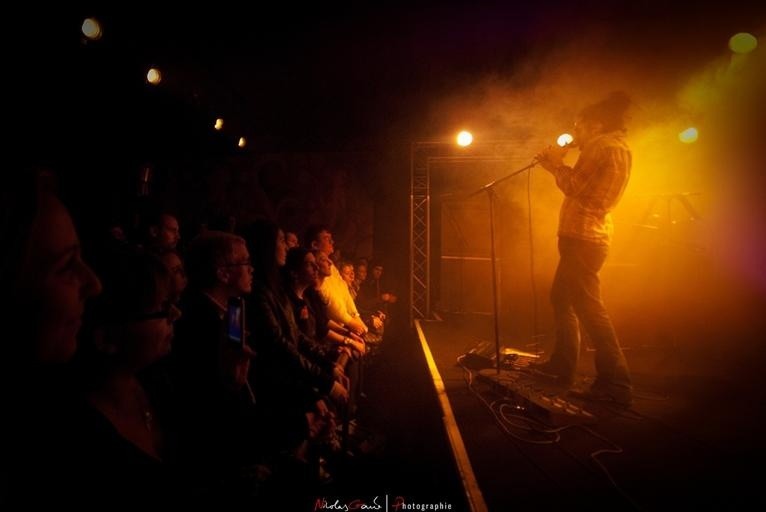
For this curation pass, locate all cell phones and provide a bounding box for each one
[227,295,245,359]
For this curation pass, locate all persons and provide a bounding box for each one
[1,178,399,511]
[531,92,636,406]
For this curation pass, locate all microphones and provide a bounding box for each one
[562,139,579,150]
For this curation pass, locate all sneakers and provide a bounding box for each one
[568,384,632,403]
[523,361,573,383]
